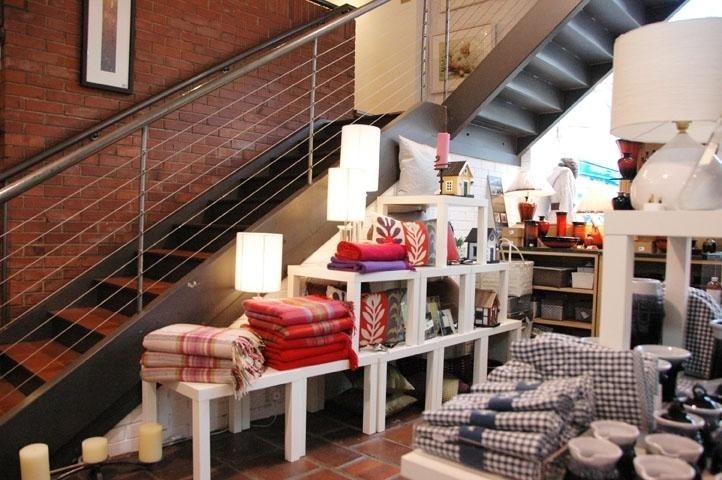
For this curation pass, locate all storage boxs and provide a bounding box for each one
[532,265,594,323]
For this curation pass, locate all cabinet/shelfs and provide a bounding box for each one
[495,248,599,337]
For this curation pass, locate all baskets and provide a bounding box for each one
[540,261,597,326]
[475,238,534,297]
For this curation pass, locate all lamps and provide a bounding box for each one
[325,122,381,242]
[607,15,722,210]
[504,166,557,222]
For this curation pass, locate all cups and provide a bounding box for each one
[564,417,708,480]
[633,345,692,406]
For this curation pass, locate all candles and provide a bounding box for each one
[18,421,165,480]
[435,132,450,168]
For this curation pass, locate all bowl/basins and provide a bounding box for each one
[540,236,580,248]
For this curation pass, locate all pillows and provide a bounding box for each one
[396,133,441,194]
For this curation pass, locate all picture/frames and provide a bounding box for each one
[428,21,498,96]
[79,0,137,97]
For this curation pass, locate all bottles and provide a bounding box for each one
[537,216,550,236]
[555,211,567,237]
[572,222,586,244]
[652,384,722,473]
[523,219,539,248]
[705,277,721,306]
